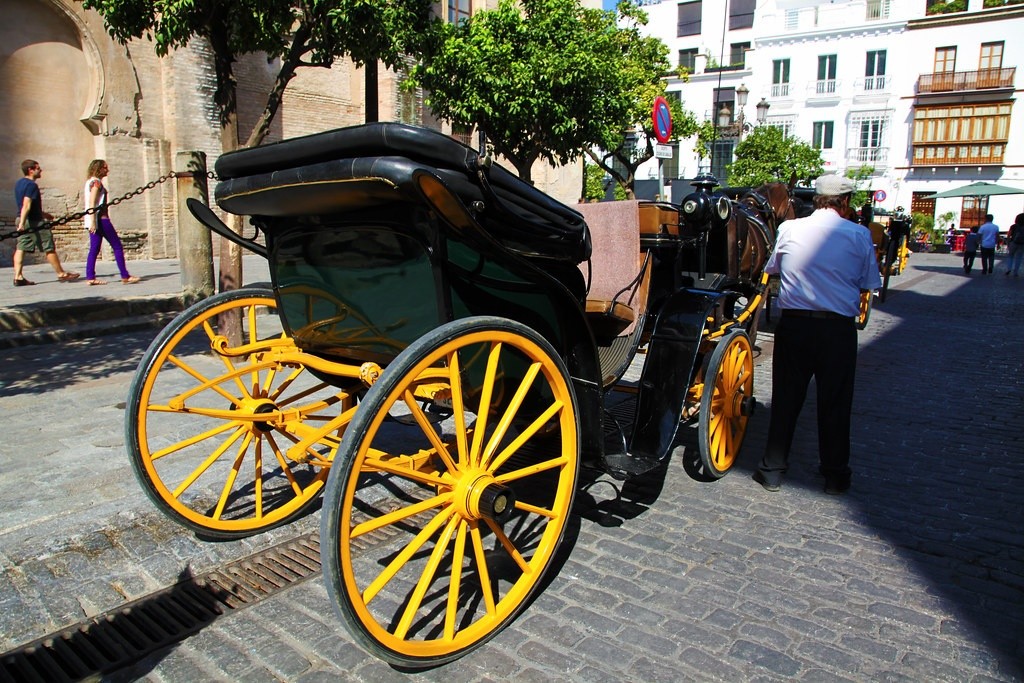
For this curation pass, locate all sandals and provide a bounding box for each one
[87,279,107,285]
[121,276,140,284]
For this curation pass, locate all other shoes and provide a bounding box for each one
[826,485,846,495]
[988,267,992,273]
[1005,268,1011,275]
[754,469,781,491]
[982,270,986,274]
[1014,273,1019,276]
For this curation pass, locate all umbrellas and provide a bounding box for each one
[921,182,1024,250]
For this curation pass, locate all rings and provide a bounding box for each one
[94,229,95,231]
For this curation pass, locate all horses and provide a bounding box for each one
[702,170,799,352]
[868,222,888,274]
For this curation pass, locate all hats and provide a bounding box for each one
[814,174,856,194]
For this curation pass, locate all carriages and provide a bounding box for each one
[121,117,912,677]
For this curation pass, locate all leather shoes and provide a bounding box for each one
[58,272,80,282]
[14,277,35,286]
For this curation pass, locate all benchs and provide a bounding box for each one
[214,122,884,392]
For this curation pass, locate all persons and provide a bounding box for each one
[946,224,956,251]
[1003,213,1024,276]
[753,174,885,495]
[84,160,141,286]
[963,226,979,274]
[13,160,80,287]
[977,214,1001,275]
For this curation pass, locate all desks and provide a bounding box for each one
[942,234,954,250]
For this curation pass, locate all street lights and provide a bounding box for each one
[734,82,772,147]
